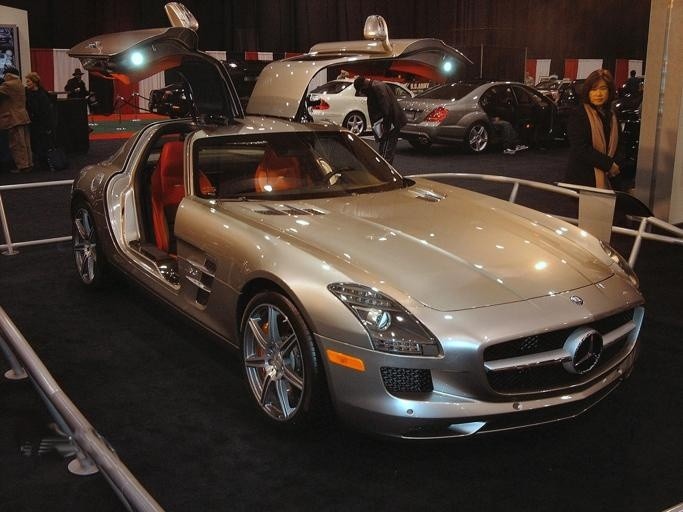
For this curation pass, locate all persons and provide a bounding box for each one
[522,71,535,88]
[0,66,90,174]
[573,68,628,192]
[628,70,638,83]
[353,75,408,166]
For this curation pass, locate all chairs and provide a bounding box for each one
[254,155,315,194]
[147,139,217,261]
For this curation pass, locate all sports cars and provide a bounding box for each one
[65,1,646,444]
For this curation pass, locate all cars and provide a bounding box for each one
[148,58,273,119]
[530,75,644,167]
[305,77,414,137]
[396,82,575,153]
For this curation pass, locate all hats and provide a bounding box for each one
[73,69,84,76]
[3,67,19,76]
[354,77,361,96]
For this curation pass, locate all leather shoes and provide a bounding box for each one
[10,167,32,174]
